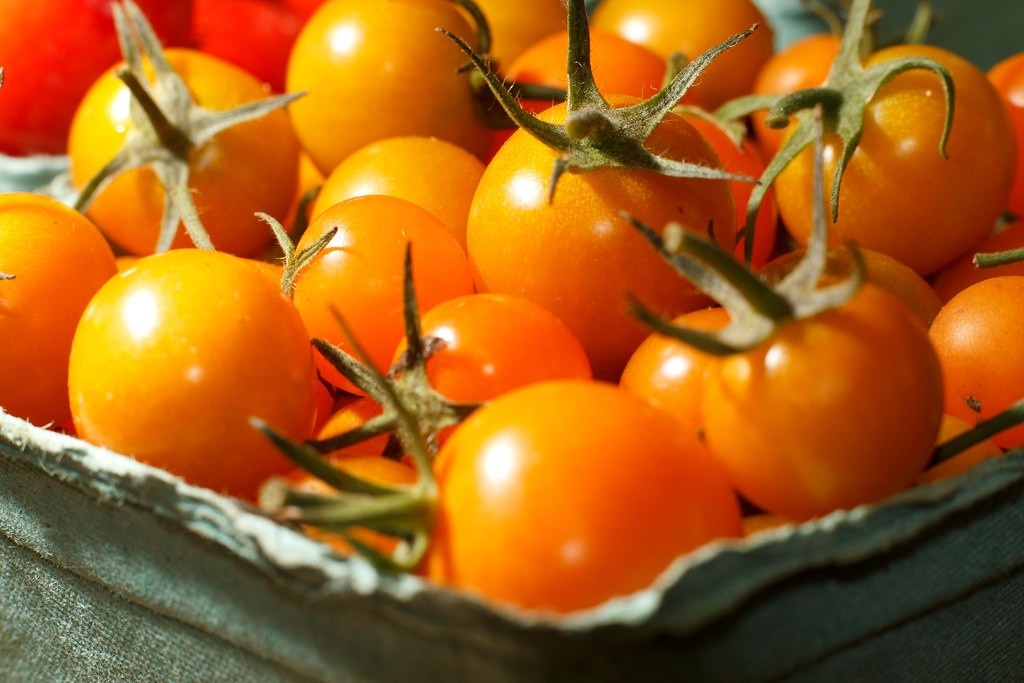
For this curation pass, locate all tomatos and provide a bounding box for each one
[3,0,1024,616]
